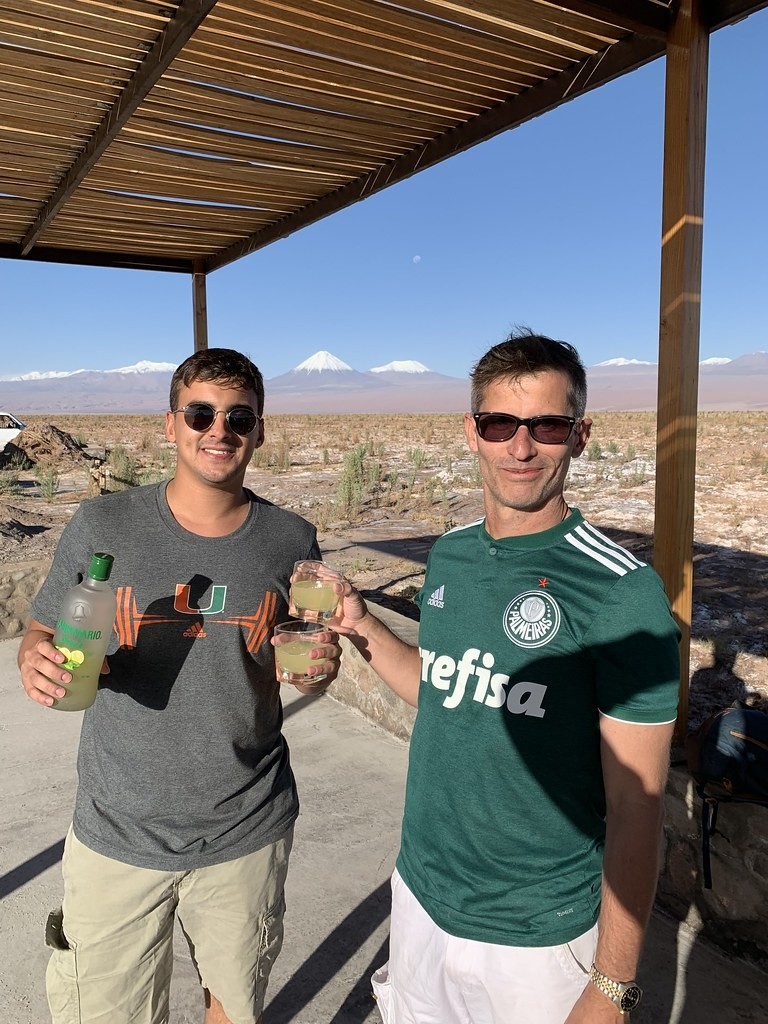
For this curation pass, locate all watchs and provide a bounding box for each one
[586,963,643,1014]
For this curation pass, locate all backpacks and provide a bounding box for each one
[686,700,768,891]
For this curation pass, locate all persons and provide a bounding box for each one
[286,327,682,1022]
[16,345,349,1024]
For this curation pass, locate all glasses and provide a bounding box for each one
[473,411,581,444]
[173,402,262,437]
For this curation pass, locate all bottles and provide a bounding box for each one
[47,552,116,711]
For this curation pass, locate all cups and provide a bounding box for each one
[274,621,327,685]
[290,560,344,622]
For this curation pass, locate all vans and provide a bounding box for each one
[0,413,27,452]
